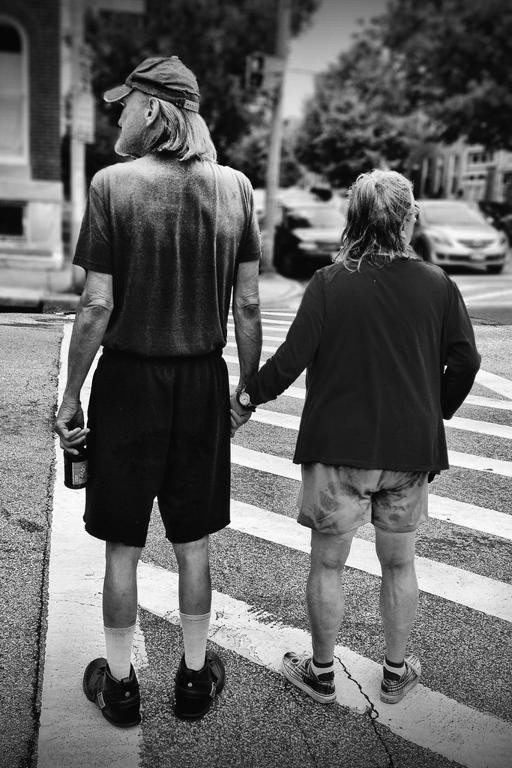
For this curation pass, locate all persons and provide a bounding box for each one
[50,53,262,730]
[230,166,482,707]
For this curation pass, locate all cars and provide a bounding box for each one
[250,188,350,278]
[410,200,508,274]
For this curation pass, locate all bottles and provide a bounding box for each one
[64,444,90,489]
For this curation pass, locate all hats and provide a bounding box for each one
[102,56,201,113]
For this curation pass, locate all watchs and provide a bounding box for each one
[237,386,258,413]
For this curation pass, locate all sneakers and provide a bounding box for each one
[82,657,141,727]
[379,654,421,703]
[283,652,337,704]
[176,652,224,720]
